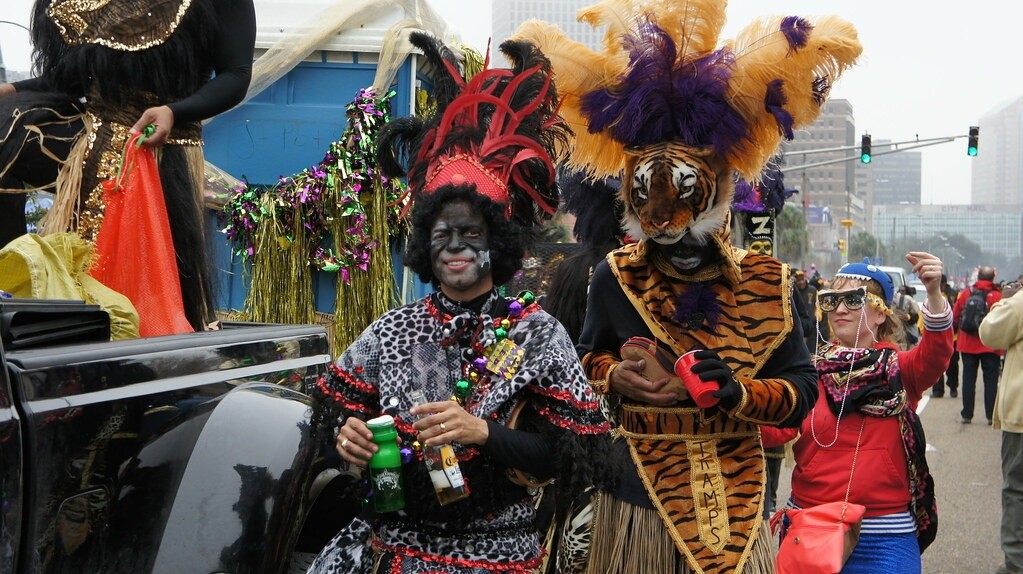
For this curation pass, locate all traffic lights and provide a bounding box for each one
[967,125,979,156]
[860,134,872,164]
[838,240,845,251]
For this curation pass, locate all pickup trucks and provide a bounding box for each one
[0,298,352,574]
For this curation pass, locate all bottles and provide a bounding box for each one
[410,389,470,505]
[366,415,405,514]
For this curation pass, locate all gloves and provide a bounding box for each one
[690,350,743,413]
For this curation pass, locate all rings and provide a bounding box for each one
[341,439,348,448]
[439,423,446,433]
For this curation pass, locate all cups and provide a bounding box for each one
[674,349,720,407]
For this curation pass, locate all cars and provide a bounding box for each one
[906,279,929,311]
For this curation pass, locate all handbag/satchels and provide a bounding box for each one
[774,502,865,574]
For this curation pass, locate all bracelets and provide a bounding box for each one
[924,295,947,312]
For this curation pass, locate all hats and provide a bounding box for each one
[510,0,860,183]
[376,27,560,232]
[730,156,800,244]
[835,262,894,307]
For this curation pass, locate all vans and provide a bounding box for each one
[876,265,910,308]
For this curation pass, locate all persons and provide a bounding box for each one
[977,274,1023,574]
[892,266,1006,426]
[0,0,256,338]
[755,251,955,574]
[544,141,820,574]
[283,147,608,574]
[788,263,830,351]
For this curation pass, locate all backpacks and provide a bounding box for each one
[958,285,996,337]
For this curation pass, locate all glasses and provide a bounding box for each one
[816,286,893,317]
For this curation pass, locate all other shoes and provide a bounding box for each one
[929,392,942,397]
[950,388,957,397]
[961,417,971,423]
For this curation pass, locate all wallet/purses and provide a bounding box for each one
[620,336,688,401]
